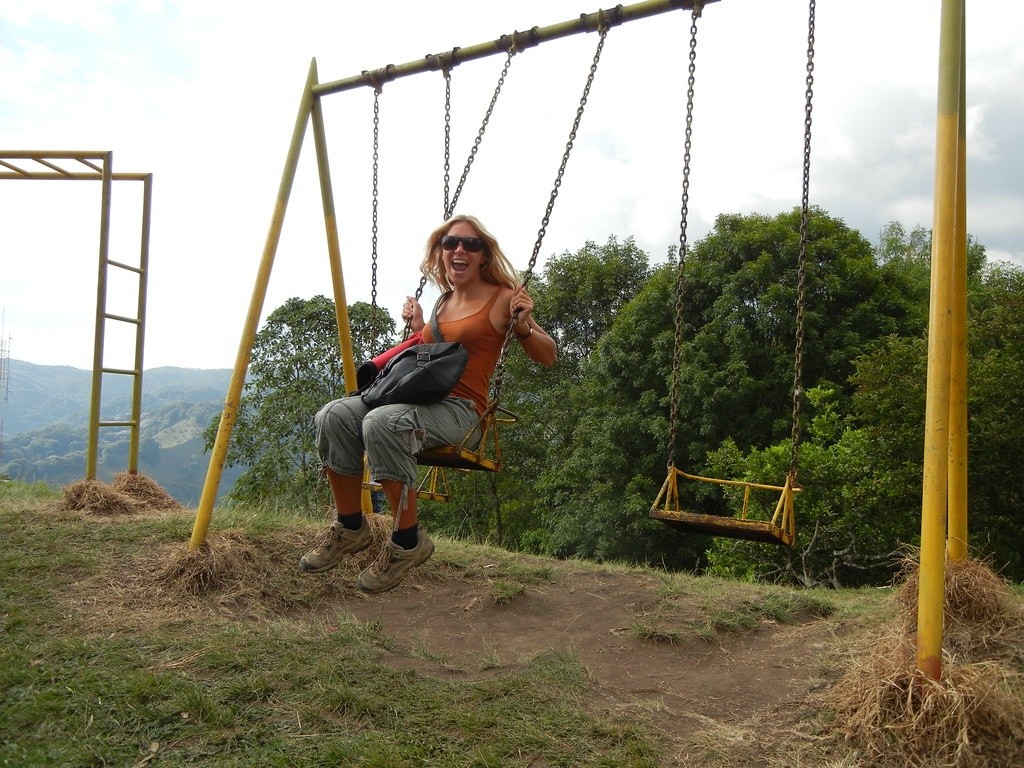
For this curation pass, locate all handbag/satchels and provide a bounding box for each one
[361,342,468,409]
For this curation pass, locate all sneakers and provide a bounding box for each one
[299,514,371,573]
[357,522,435,594]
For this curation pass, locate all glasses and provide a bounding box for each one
[441,236,484,253]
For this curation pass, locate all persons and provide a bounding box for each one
[297,214,556,593]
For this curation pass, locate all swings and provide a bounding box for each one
[401,24,610,473]
[648,0,815,548]
[361,76,452,504]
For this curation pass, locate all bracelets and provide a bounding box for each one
[518,322,533,338]
